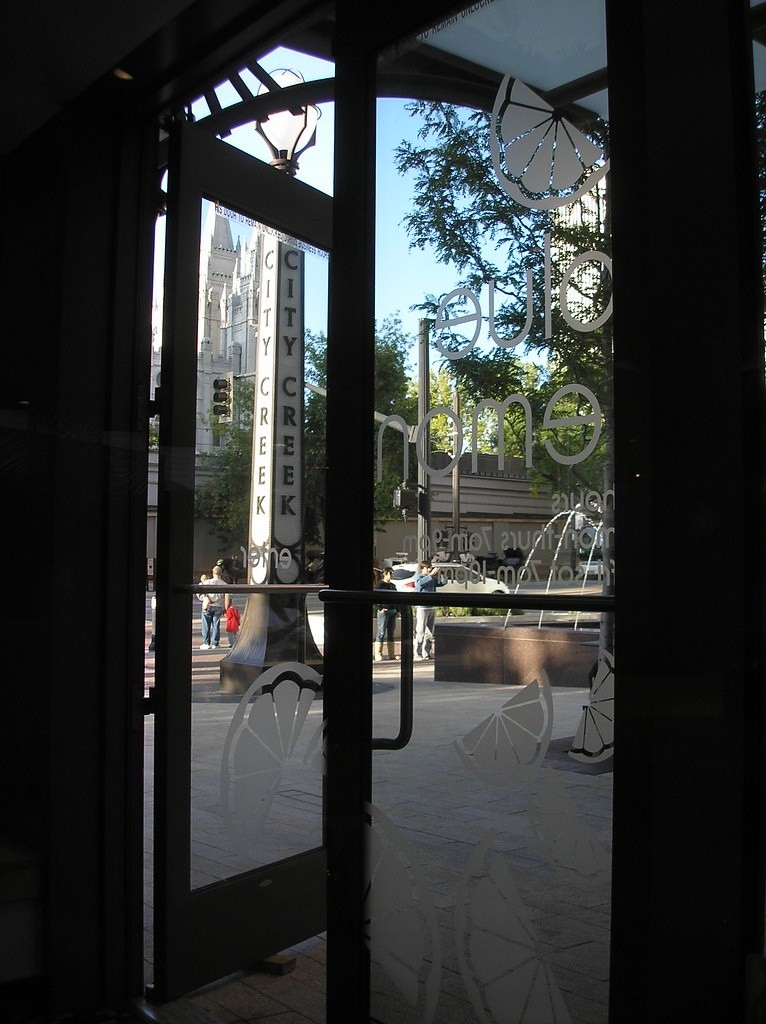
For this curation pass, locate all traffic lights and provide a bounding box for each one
[212,371,234,424]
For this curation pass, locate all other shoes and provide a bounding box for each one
[413,657,423,662]
[212,646,220,649]
[229,646,232,648]
[200,644,210,650]
[423,657,430,661]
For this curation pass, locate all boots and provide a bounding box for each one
[375,642,384,661]
[387,642,401,660]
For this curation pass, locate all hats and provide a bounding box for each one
[211,566,222,575]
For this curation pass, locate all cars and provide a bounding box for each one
[390,560,511,594]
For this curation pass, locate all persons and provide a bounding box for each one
[196,555,239,650]
[413,560,448,662]
[374,567,401,661]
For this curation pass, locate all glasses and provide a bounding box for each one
[387,571,393,574]
